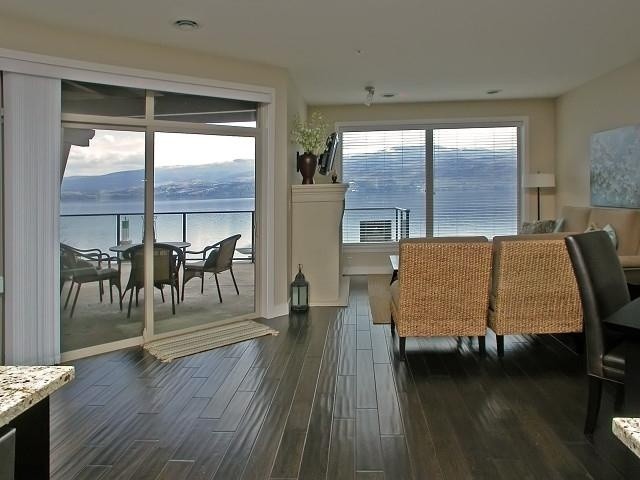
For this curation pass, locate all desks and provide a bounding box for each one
[610,417,640,459]
[108,241,190,308]
[599,295,640,418]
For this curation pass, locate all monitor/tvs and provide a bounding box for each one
[318,132,339,175]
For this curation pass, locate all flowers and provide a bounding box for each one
[290,107,333,156]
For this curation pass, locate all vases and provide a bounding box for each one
[298,152,317,184]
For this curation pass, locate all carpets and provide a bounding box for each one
[365,274,393,323]
[141,318,279,363]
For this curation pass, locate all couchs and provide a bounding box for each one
[555,203,640,290]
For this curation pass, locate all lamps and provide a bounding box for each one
[520,170,557,221]
[289,262,311,314]
[364,86,375,106]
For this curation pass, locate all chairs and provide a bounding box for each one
[485,231,589,360]
[387,234,491,363]
[565,229,629,440]
[180,234,242,304]
[59,243,104,304]
[64,250,125,318]
[120,243,180,319]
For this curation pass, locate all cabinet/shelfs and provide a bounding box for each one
[0,365,76,480]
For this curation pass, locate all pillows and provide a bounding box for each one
[519,220,556,235]
[582,221,618,255]
